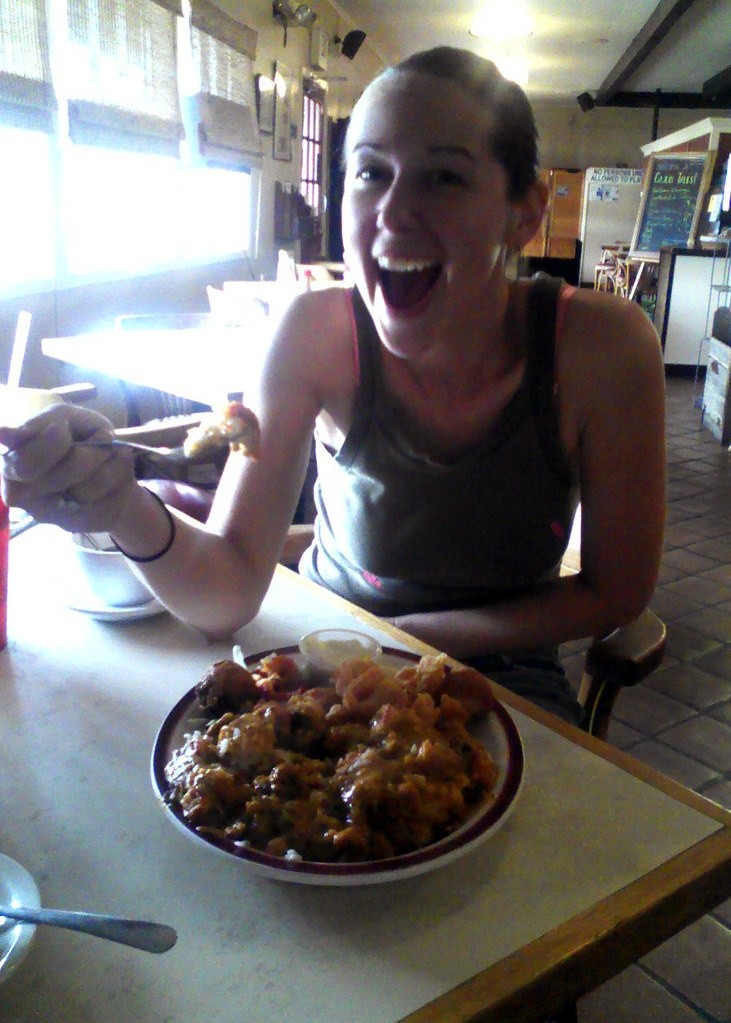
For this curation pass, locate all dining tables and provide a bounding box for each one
[0,446,731,1023]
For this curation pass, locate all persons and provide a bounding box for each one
[0,48,669,738]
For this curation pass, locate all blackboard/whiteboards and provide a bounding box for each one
[628,151,716,262]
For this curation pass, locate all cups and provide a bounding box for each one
[67,529,156,606]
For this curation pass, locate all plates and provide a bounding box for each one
[0,854,43,989]
[63,585,166,623]
[150,646,526,886]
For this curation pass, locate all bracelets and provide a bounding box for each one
[108,485,176,562]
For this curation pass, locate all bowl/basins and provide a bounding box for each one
[299,629,383,684]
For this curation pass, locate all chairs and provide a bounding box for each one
[270,519,669,744]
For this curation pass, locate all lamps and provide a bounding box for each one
[575,89,601,113]
[271,0,317,47]
[335,30,372,60]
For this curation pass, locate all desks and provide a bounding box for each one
[592,241,657,303]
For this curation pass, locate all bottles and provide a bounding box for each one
[302,270,312,293]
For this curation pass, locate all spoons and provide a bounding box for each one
[69,426,251,463]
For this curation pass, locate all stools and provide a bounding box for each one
[594,263,616,295]
[606,270,628,298]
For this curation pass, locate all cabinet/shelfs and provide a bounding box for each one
[520,169,583,259]
[693,235,731,413]
